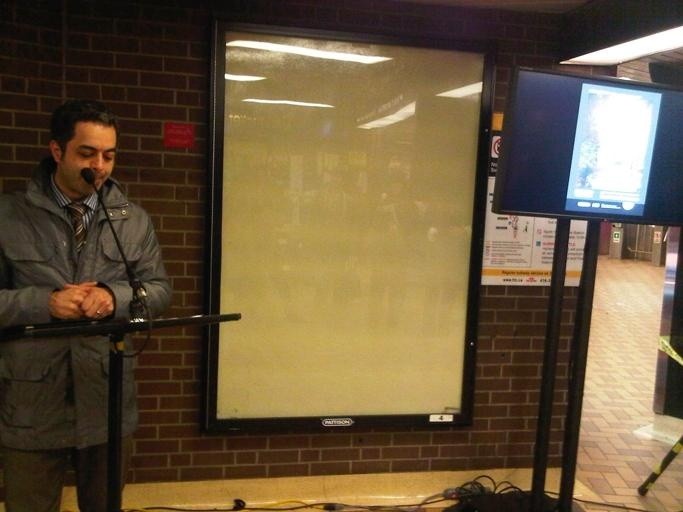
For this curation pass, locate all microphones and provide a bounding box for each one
[80,167,150,320]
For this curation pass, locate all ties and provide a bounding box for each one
[69,203,87,257]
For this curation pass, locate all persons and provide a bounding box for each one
[0,100,170,512]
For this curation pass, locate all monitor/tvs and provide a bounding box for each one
[492,65,683,227]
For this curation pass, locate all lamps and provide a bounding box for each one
[559,0,683,65]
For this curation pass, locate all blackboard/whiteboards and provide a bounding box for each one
[209,13,499,432]
